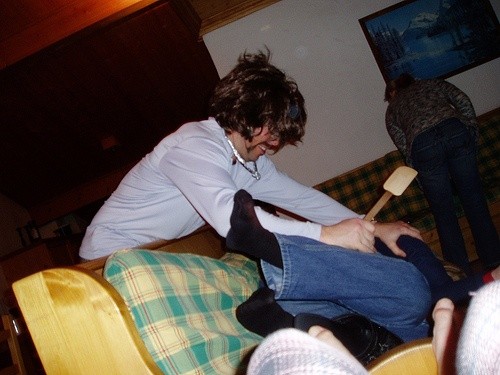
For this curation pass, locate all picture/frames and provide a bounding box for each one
[359,0,500,85]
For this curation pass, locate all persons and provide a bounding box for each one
[224,184,499,374]
[72,43,425,268]
[384,70,500,271]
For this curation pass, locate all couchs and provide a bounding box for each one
[310,107,500,263]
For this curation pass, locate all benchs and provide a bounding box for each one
[12,222,435,375]
